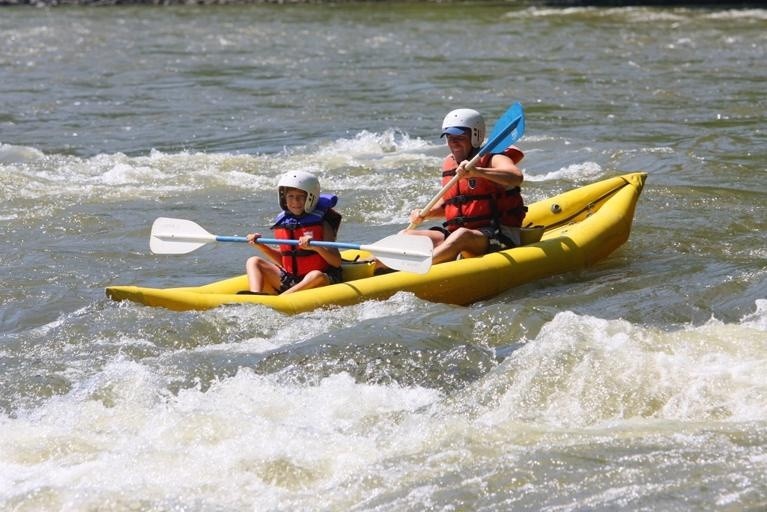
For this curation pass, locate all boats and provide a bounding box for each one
[104,170,648,320]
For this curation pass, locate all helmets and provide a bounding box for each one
[276,169,319,214]
[438,108,485,149]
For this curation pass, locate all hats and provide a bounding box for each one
[440,127,465,139]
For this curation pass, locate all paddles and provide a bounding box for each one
[149,217,434,274]
[406,101,526,229]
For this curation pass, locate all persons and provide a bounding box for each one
[372,108,525,274]
[244,171,344,295]
